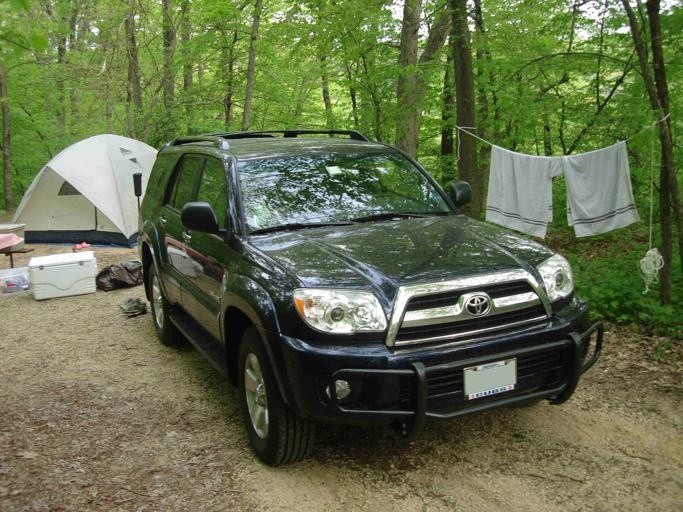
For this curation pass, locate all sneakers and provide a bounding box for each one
[120,298,146,312]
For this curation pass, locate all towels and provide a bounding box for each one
[564,139,641,239]
[484,145,554,239]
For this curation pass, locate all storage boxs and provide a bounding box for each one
[0,267,30,293]
[0,223,26,244]
[29,251,97,301]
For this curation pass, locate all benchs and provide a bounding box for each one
[0,248,34,268]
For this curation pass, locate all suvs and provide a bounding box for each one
[141,130,603,466]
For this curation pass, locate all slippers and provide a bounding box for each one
[75,242,91,250]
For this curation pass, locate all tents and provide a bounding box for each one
[12,132,161,250]
